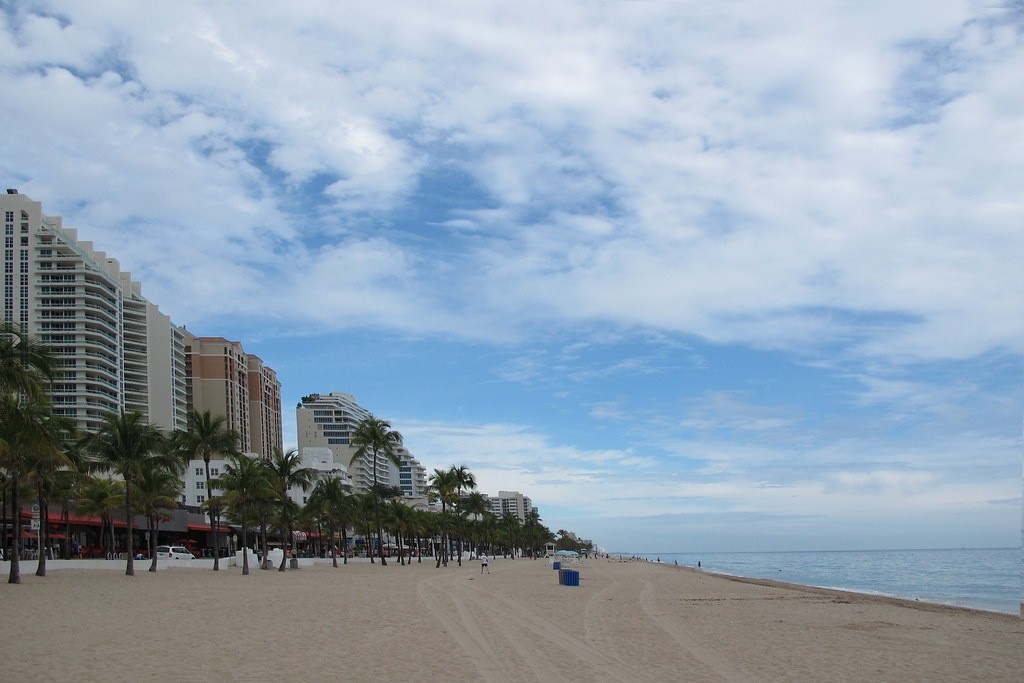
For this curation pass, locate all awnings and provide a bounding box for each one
[187,523,321,541]
[21,511,139,528]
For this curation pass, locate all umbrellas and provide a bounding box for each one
[6,528,71,560]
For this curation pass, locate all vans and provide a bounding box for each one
[156,544,197,560]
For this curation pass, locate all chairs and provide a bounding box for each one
[26,549,35,560]
[33,550,40,559]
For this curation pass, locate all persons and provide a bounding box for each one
[286,542,347,558]
[77,543,83,560]
[481,553,490,574]
[72,541,78,559]
[527,547,702,571]
